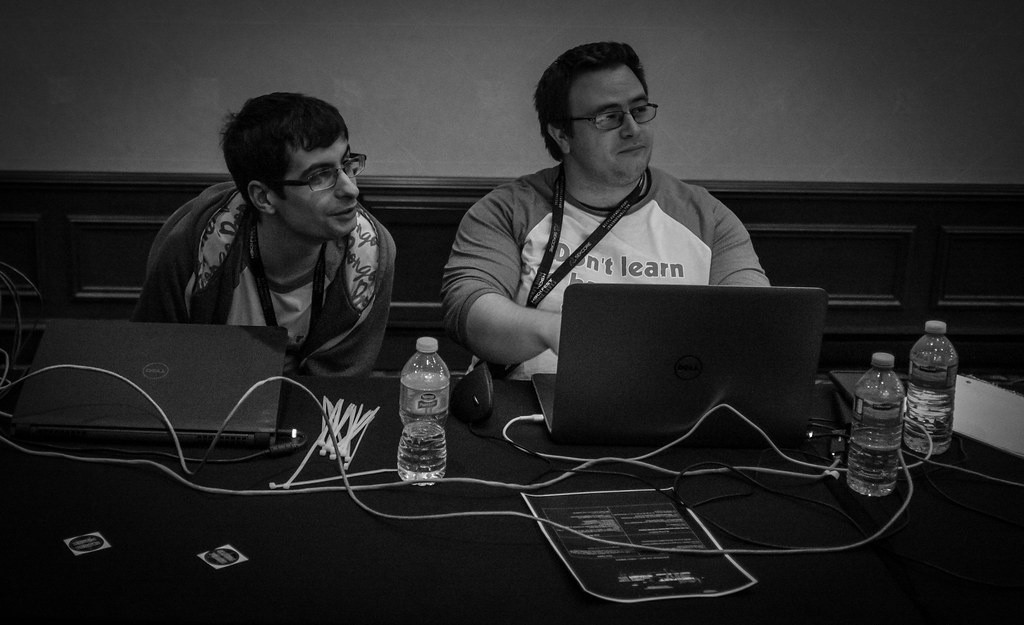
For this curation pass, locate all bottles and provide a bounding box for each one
[846,352,905,497]
[903,320,958,455]
[397,337,451,487]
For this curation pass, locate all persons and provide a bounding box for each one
[131,93,398,386]
[439,42,772,381]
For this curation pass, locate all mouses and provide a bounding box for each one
[450,367,494,428]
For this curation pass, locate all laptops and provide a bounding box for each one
[14,320,289,449]
[532,284,829,443]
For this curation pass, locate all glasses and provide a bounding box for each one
[570,101,659,131]
[283,153,367,191]
